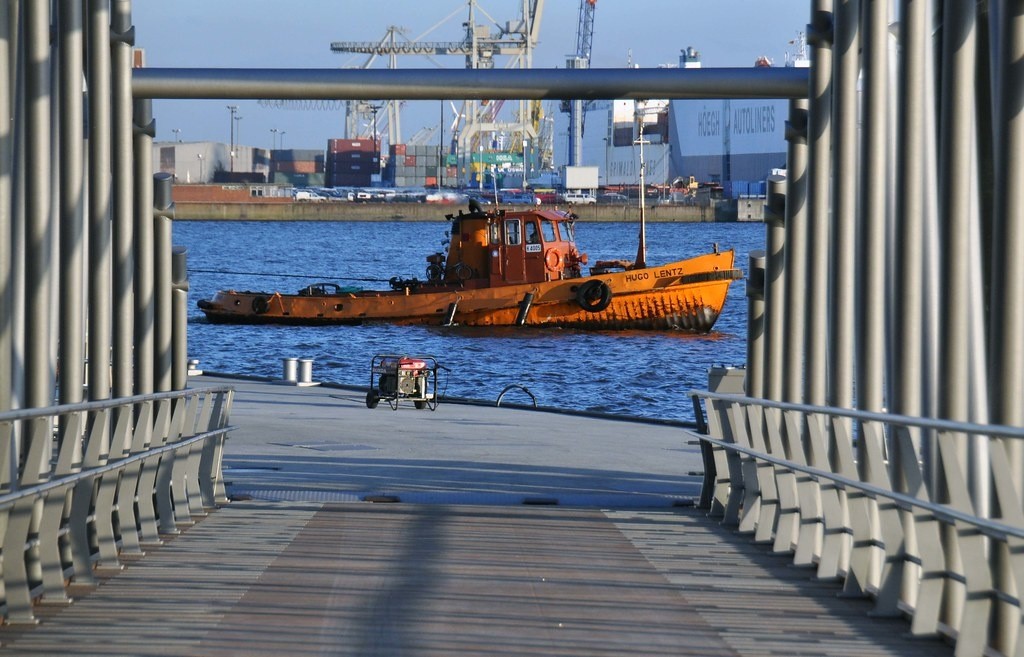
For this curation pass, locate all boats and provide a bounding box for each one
[196,208,744,336]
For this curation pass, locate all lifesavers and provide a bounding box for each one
[545,248,563,270]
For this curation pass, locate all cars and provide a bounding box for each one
[294,176,726,205]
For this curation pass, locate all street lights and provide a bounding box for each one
[227,105,237,183]
[603,137,611,186]
[270,128,277,162]
[368,105,384,159]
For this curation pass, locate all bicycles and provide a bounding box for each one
[425,245,473,282]
